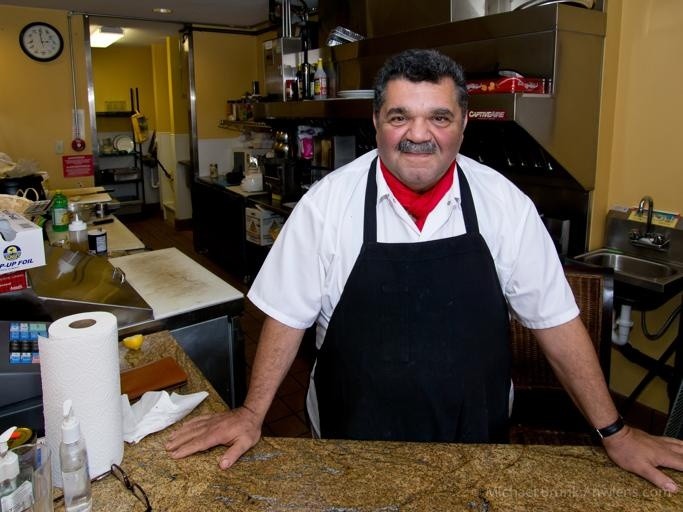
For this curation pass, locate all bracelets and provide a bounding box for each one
[594,413,626,440]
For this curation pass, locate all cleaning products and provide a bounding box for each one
[50,189,69,232]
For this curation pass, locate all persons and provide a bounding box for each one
[164,47,683,494]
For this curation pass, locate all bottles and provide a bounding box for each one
[226,79,262,122]
[49,189,107,256]
[209,163,219,179]
[286,58,328,100]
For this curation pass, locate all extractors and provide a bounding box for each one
[264,5,606,193]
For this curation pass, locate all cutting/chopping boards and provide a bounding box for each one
[57,185,112,203]
[85,213,145,251]
[108,246,245,321]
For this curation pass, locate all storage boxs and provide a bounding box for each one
[115,196,143,215]
[244,202,283,246]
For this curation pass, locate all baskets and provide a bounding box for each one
[0,187,39,215]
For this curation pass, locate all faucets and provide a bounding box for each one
[636,196,653,236]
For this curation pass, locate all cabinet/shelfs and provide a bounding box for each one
[191,178,250,287]
[95,87,147,215]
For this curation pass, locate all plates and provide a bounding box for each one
[335,90,374,99]
[113,134,135,153]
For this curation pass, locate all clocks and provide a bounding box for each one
[19,21,65,62]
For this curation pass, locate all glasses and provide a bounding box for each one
[53,463,152,512]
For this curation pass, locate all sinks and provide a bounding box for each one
[564,246,682,312]
